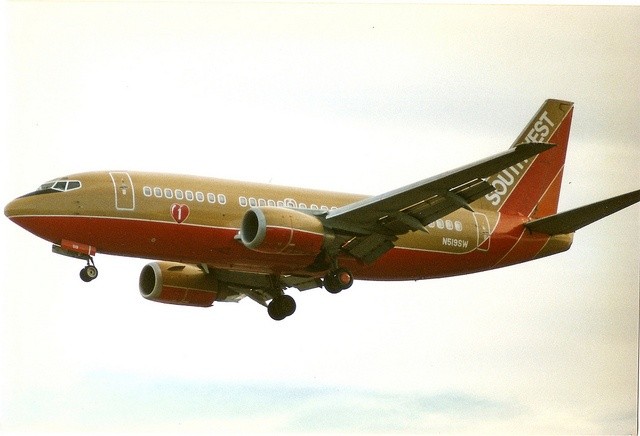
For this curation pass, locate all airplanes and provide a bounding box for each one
[4,99,640,321]
